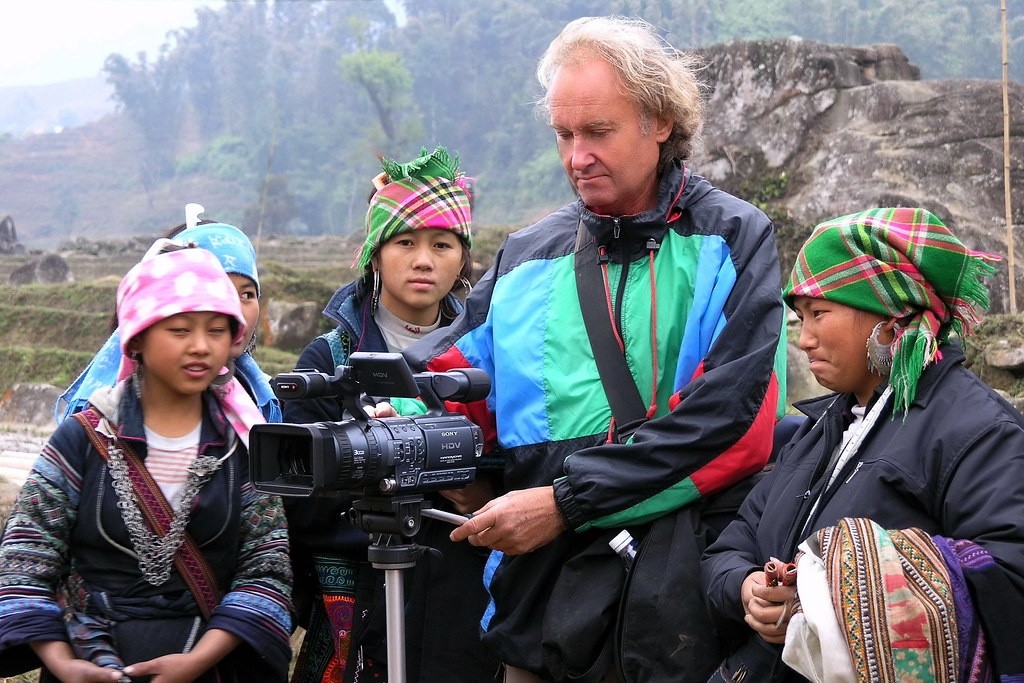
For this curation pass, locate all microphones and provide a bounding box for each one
[432,368,491,403]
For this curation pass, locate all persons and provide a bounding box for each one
[696,208,1024,683]
[1,14,787,683]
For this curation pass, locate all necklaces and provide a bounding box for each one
[91,412,240,588]
